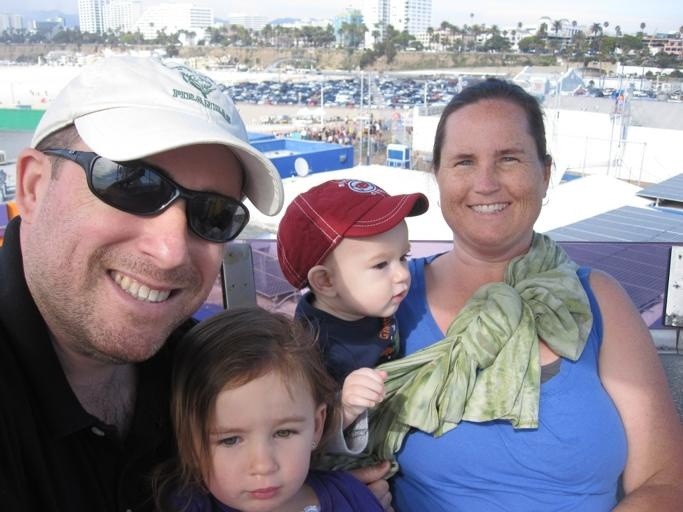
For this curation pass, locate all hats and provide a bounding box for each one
[276,177,429,289]
[29,54,285,217]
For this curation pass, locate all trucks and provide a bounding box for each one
[633,91,646,96]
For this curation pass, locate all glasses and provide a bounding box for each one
[42,148,250,244]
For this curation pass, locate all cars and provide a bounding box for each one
[218,77,459,108]
[590,88,624,99]
[671,92,681,100]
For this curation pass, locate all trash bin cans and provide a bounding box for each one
[387,143,412,169]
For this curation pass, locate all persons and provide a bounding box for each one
[308,75,683,512]
[0,52,286,511]
[135,302,384,511]
[276,177,431,476]
